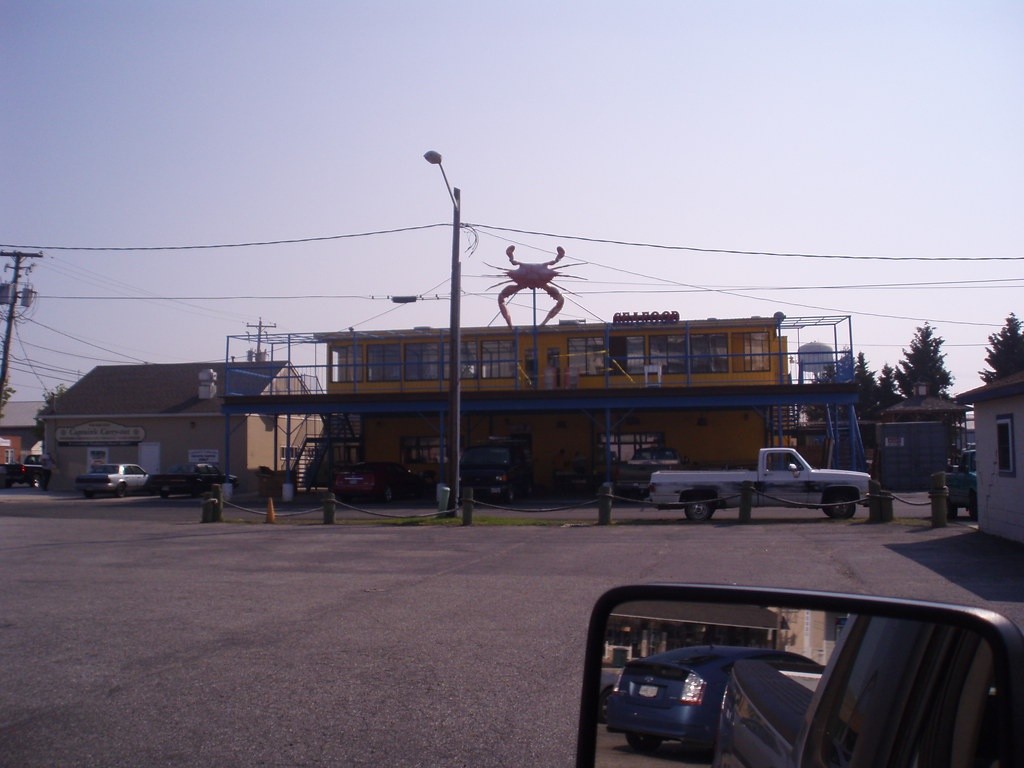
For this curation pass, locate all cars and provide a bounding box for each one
[74,462,150,499]
[606,643,826,762]
[148,462,239,498]
[334,460,425,505]
[597,668,619,725]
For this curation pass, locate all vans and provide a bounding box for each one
[448,437,534,504]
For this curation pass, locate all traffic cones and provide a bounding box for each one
[262,498,278,526]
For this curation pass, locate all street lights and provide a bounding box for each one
[422,151,461,516]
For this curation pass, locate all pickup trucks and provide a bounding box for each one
[0,453,45,488]
[944,448,978,519]
[648,445,872,525]
[615,445,681,491]
